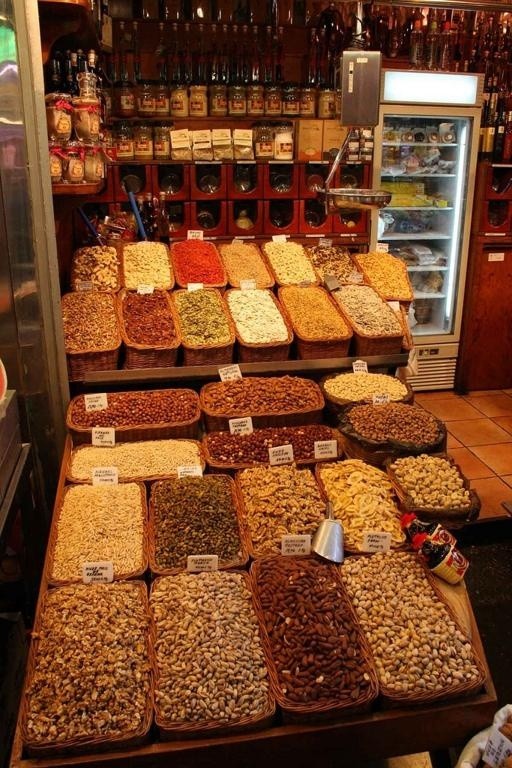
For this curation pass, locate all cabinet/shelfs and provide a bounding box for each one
[9,379,512,768]
[37,0,512,392]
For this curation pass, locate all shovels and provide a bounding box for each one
[312,501,343,563]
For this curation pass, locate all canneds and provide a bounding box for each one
[113,120,134,161]
[155,78,170,118]
[264,80,284,117]
[272,120,295,160]
[298,80,317,120]
[151,120,175,160]
[245,80,265,118]
[131,120,154,160]
[317,83,337,120]
[188,79,209,118]
[114,80,137,119]
[281,81,301,118]
[136,78,156,118]
[227,81,248,118]
[252,120,275,160]
[101,88,113,124]
[170,78,190,118]
[209,79,229,119]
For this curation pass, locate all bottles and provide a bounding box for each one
[400,513,459,549]
[48,46,101,97]
[219,24,230,83]
[263,25,274,83]
[118,19,128,83]
[410,532,470,586]
[439,21,452,70]
[250,25,261,83]
[425,21,438,70]
[274,26,284,83]
[463,13,511,77]
[409,19,425,68]
[240,25,250,83]
[308,27,317,84]
[319,28,328,84]
[208,24,220,83]
[171,21,182,82]
[130,21,144,82]
[365,5,405,58]
[231,25,239,84]
[182,22,193,83]
[196,22,208,84]
[478,77,512,164]
[157,21,168,81]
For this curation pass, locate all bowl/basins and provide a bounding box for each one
[317,188,393,211]
